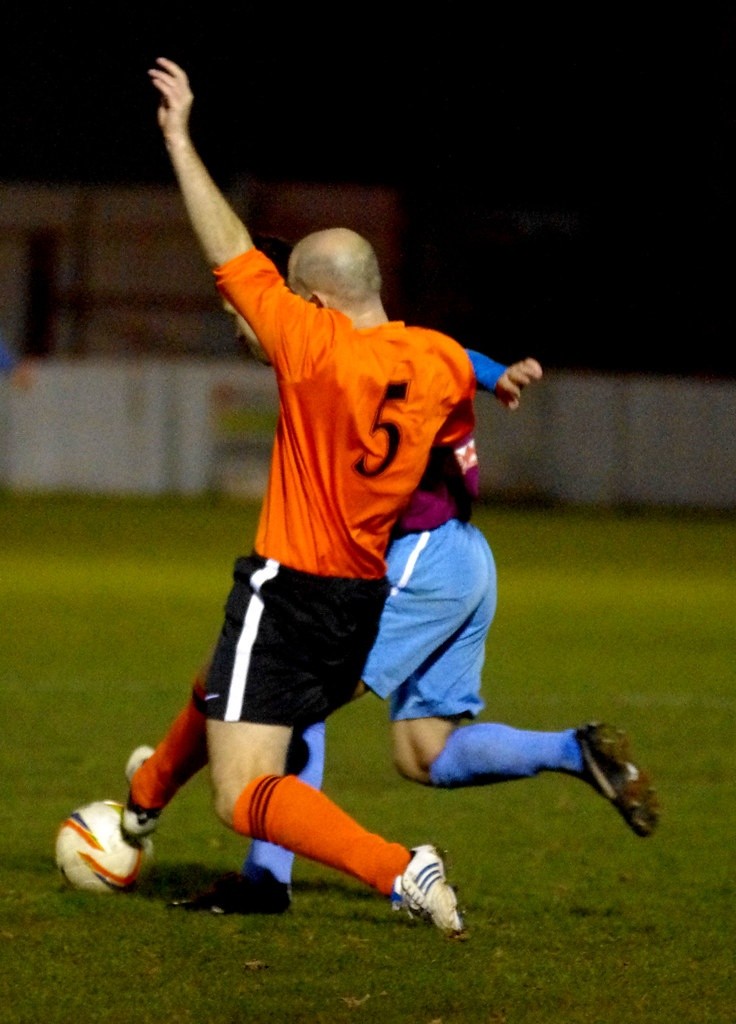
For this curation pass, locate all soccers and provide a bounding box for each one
[55,799,155,895]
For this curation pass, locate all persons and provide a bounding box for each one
[122,57,477,941]
[167,233,660,914]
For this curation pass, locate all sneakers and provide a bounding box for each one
[165,870,292,916]
[574,723,661,837]
[121,744,161,837]
[391,844,460,938]
